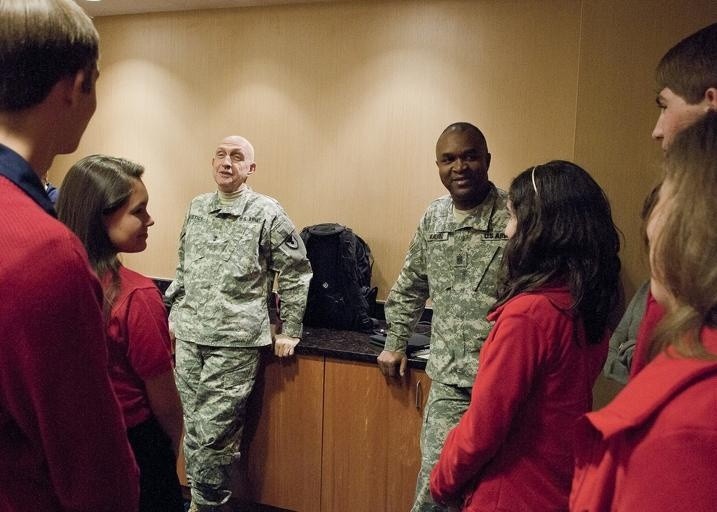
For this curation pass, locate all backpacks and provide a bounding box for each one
[299,222,378,333]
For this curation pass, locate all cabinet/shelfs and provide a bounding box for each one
[321,354,434,512]
[230,353,326,512]
